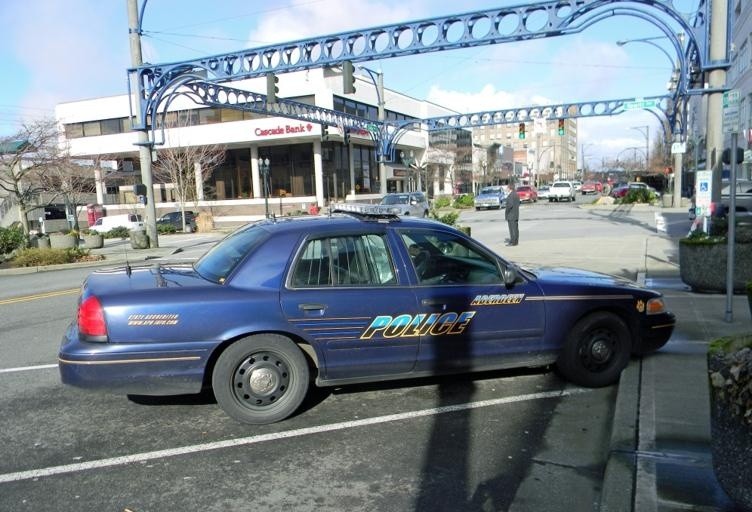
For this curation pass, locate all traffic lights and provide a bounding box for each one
[342,59,357,96]
[519,123,525,139]
[343,125,351,145]
[321,123,328,143]
[266,72,279,104]
[558,119,564,136]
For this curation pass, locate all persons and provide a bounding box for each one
[336,235,420,277]
[504,182,519,246]
[309,202,319,215]
[686,216,700,238]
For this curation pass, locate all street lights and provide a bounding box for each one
[358,63,387,193]
[258,157,274,220]
[615,29,689,208]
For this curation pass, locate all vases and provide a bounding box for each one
[38,238,50,249]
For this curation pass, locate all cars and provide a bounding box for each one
[154,210,197,232]
[515,186,537,203]
[61,205,679,425]
[535,181,603,203]
[471,186,504,212]
[610,181,661,201]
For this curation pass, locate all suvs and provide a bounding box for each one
[690,174,752,217]
[366,191,429,221]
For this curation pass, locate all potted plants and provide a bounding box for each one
[427,209,471,257]
[49,230,78,249]
[84,229,103,248]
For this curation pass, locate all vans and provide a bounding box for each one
[88,213,146,234]
